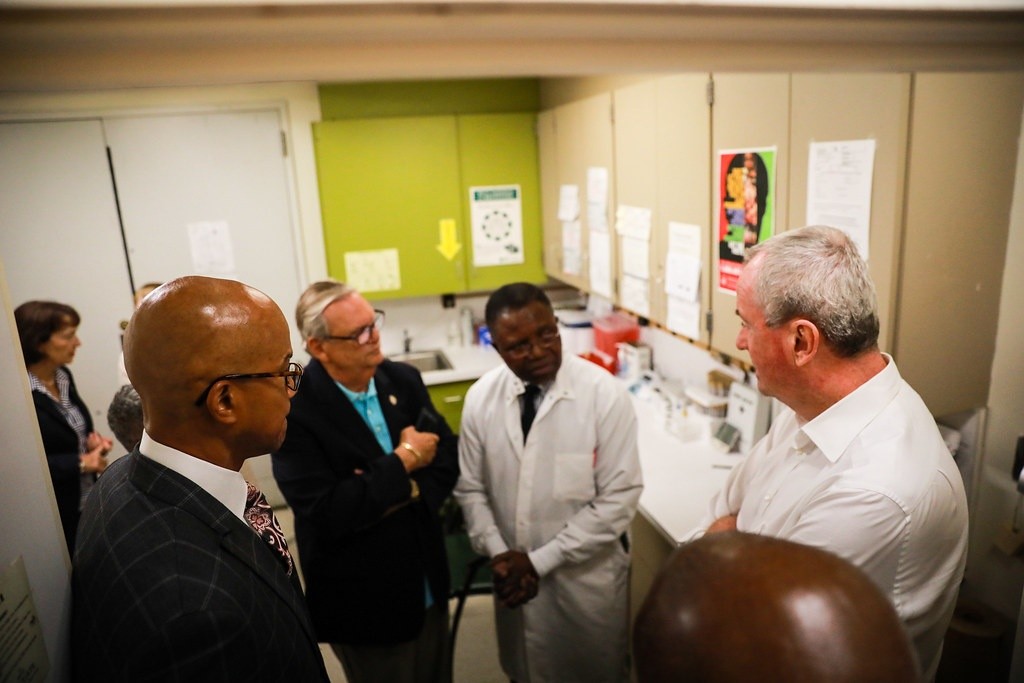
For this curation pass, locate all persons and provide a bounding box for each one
[14,300,113,563]
[108,384,146,451]
[272,281,459,683]
[678,224,970,683]
[629,529,923,683]
[68,273,331,683]
[460,281,642,683]
[118,281,164,383]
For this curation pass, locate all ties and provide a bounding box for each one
[521,384,540,445]
[244,482,294,580]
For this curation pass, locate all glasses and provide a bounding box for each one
[324,308,386,346]
[509,328,561,360]
[195,363,305,408]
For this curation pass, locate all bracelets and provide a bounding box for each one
[400,442,421,466]
[79,454,86,475]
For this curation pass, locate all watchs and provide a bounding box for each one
[409,479,421,503]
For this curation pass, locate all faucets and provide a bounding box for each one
[402,328,415,353]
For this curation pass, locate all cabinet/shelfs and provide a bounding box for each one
[311,111,469,305]
[479,310,756,679]
[417,376,481,437]
[2,99,297,683]
[457,112,552,294]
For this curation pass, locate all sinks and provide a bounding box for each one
[384,348,455,372]
